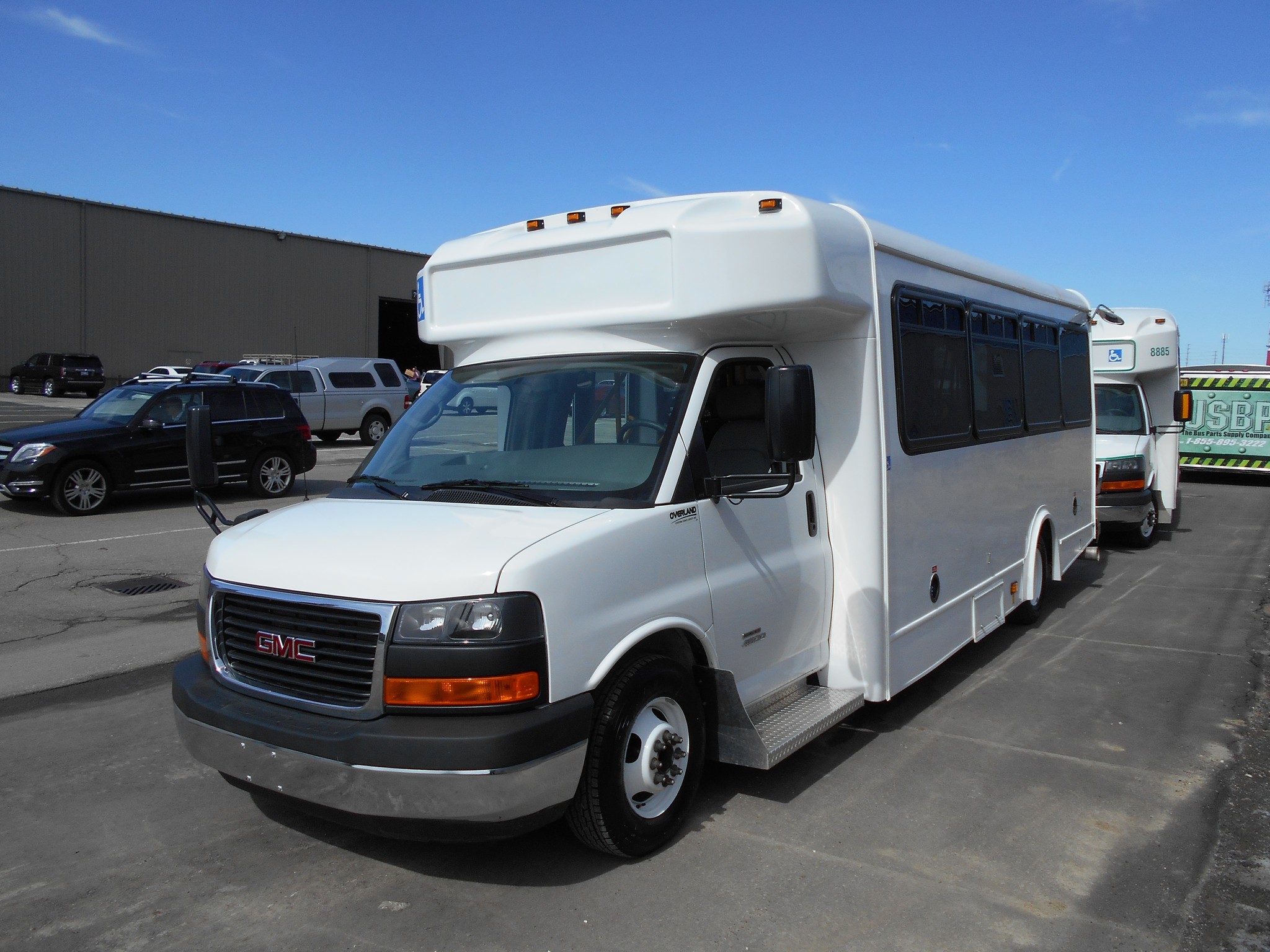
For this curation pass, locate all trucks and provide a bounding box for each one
[1081,307,1194,548]
[162,190,1096,863]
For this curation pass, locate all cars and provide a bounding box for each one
[134,365,192,383]
[402,374,422,400]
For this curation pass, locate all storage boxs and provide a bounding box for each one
[405,369,415,377]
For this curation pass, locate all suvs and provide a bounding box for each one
[0,373,315,514]
[419,371,500,414]
[8,352,106,398]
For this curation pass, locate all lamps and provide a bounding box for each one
[277,232,287,240]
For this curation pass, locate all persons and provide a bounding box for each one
[158,396,187,423]
[408,366,426,382]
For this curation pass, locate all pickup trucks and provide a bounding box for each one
[218,358,411,444]
